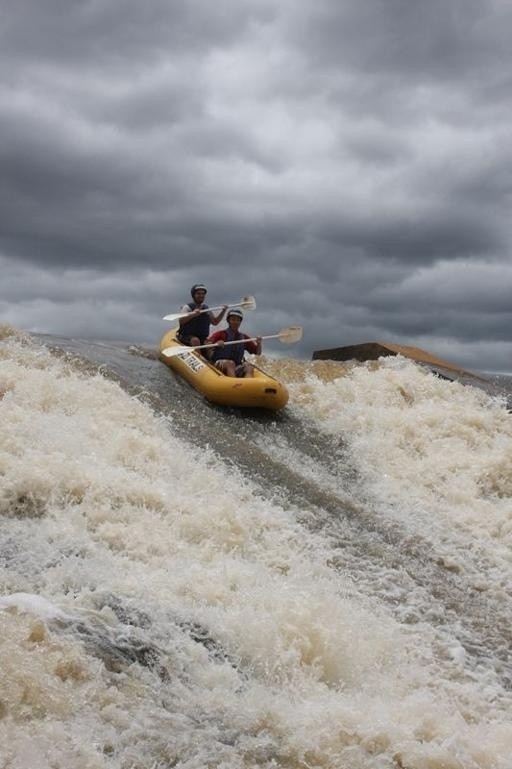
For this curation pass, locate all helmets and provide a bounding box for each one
[226,310,243,322]
[191,283,207,296]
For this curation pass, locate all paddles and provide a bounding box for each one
[163,296,256,320]
[162,327,302,357]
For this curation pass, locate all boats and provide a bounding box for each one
[157,326,291,412]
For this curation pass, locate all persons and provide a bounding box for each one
[177,283,228,355]
[206,309,263,378]
